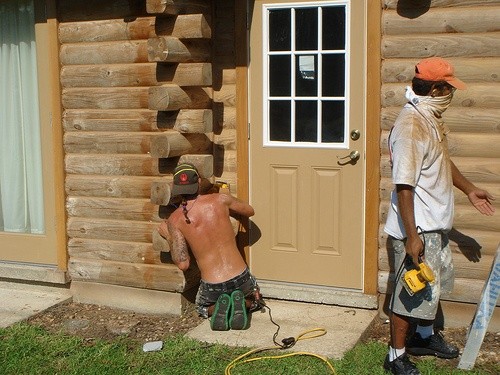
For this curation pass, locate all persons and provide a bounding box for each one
[156,163,262,331]
[381,56,496,375]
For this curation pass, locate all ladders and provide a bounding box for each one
[457,250,499,370]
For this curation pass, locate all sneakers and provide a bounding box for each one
[409,330,459,358]
[383,352,423,375]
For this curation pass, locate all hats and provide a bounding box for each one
[415,56,467,90]
[171,162,200,196]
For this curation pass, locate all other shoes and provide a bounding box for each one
[210,294,231,331]
[229,290,248,330]
